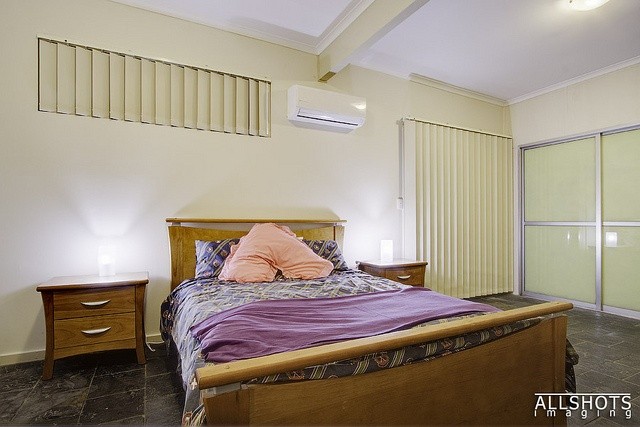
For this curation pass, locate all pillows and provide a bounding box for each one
[216,222,333,285]
[192,237,241,281]
[301,239,349,272]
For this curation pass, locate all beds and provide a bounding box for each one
[157,218,578,426]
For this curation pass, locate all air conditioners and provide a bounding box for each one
[286,84,368,131]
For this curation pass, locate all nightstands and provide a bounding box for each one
[36,269,149,381]
[355,257,430,291]
